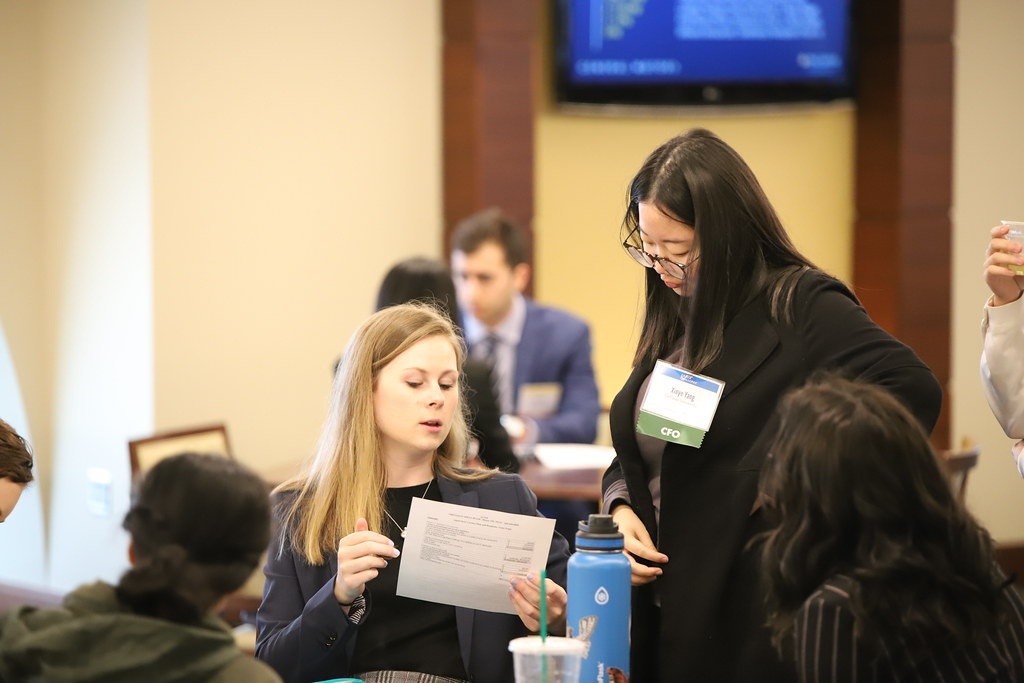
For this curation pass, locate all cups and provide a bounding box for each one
[1001,220,1024,276]
[508,636,583,683]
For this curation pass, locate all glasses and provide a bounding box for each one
[623,223,703,280]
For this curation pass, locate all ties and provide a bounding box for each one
[482,335,501,418]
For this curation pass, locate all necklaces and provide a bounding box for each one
[383,476,434,538]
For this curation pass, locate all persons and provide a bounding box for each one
[598,127,938,683]
[330,258,519,472]
[1,419,33,524]
[979,221,1023,477]
[0,451,280,682]
[432,208,597,446]
[255,301,574,682]
[745,378,1023,683]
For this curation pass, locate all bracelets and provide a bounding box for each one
[340,602,354,607]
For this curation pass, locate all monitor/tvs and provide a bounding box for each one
[547,0,856,109]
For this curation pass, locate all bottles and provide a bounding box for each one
[565,514,632,683]
